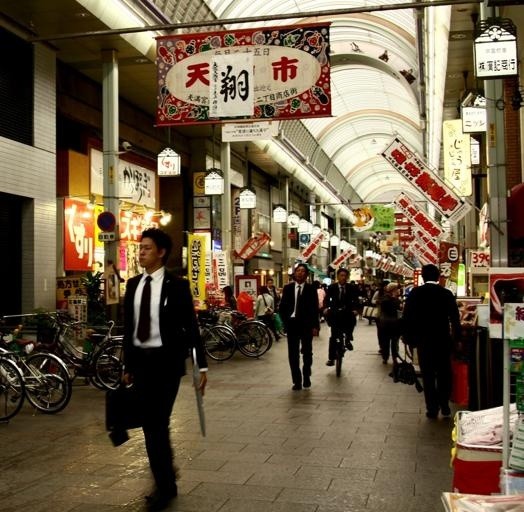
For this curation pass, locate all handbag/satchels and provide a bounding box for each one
[450,351,469,408]
[104,377,145,430]
[362,304,380,318]
[262,294,275,315]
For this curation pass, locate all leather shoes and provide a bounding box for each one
[346,340,353,351]
[327,359,335,366]
[276,336,280,341]
[293,383,302,390]
[303,375,311,387]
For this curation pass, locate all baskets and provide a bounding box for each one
[198,310,219,324]
[37,313,57,345]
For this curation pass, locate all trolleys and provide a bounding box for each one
[363,316,377,325]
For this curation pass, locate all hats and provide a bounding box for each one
[386,282,400,292]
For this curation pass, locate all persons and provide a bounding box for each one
[222,256,460,422]
[118,225,208,511]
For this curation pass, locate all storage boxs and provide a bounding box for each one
[450,408,513,497]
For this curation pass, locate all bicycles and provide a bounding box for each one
[0,311,135,424]
[190,302,287,361]
[324,306,364,377]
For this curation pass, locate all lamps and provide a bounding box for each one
[297,186,395,266]
[87,195,171,227]
[203,125,223,195]
[240,145,256,209]
[272,171,286,222]
[287,182,299,228]
[156,127,181,178]
[458,15,523,165]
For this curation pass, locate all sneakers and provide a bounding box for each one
[148,485,178,512]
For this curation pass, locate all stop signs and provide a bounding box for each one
[96,211,116,232]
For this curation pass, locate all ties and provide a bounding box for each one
[296,286,302,317]
[339,285,345,310]
[136,277,153,343]
